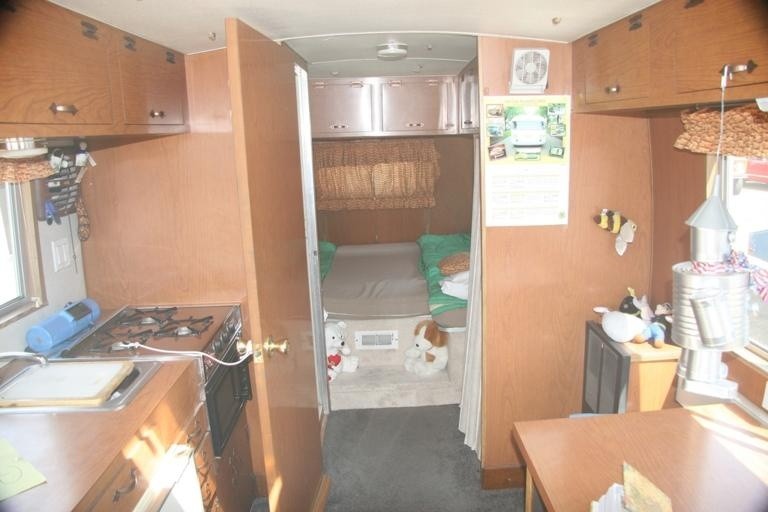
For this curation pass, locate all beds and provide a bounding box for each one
[317,241,473,368]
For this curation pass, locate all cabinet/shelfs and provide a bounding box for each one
[78,362,222,511]
[0,0,188,127]
[211,409,258,510]
[575,1,766,107]
[579,320,683,414]
[303,75,462,134]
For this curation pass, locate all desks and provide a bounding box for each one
[502,407,767,512]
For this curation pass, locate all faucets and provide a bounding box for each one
[0,348,48,369]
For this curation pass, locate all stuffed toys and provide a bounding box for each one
[324,322,360,383]
[404,320,449,377]
[635,302,673,348]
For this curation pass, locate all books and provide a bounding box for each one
[589,460,673,512]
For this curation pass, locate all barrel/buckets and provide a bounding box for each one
[670,261,750,350]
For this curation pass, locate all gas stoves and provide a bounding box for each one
[62,304,243,385]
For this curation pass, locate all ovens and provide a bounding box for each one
[204,338,252,459]
